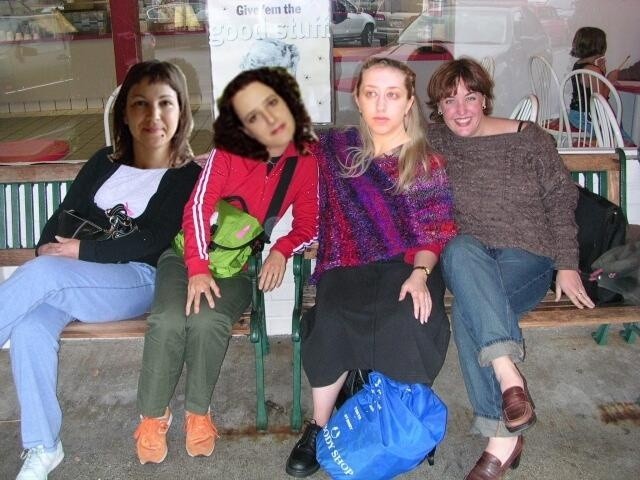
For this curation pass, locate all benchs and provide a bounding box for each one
[279,144,640,437]
[0,157,273,438]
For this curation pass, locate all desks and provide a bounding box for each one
[608,82,640,97]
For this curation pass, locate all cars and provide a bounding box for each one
[354,5,553,134]
[544,0,579,26]
[331,0,377,48]
[1,13,87,109]
[526,5,570,47]
[146,2,207,33]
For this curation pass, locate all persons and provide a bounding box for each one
[133,66,320,465]
[568,28,635,146]
[424,58,596,480]
[1,62,202,480]
[285,58,461,477]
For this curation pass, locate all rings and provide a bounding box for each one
[577,293,582,298]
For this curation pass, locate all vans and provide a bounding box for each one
[373,0,433,45]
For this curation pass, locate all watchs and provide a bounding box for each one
[413,266,432,286]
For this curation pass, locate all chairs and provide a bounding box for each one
[527,53,598,151]
[589,90,624,150]
[480,54,495,83]
[559,67,623,149]
[508,92,539,125]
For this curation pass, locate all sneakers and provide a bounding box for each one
[134,406,173,465]
[16,438,65,480]
[182,404,221,458]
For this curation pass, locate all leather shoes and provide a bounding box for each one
[285,420,322,477]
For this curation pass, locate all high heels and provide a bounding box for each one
[463,434,524,480]
[500,362,537,433]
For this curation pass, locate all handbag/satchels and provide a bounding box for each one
[55,197,140,265]
[551,177,629,307]
[315,370,448,476]
[172,195,266,279]
